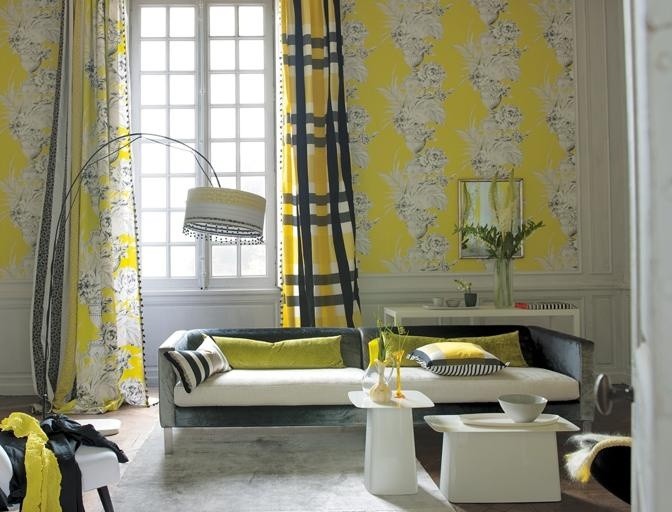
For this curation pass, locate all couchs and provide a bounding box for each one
[158,326,594,455]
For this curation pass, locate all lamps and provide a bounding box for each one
[42,134,267,439]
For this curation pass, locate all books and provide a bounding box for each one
[513,299,572,310]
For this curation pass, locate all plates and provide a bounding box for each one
[422,303,480,310]
[459,413,560,429]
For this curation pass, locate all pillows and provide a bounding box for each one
[164,333,347,393]
[380,330,527,375]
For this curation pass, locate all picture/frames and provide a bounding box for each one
[458,177,524,259]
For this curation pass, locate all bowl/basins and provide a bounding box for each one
[496,393,549,423]
[432,297,444,307]
[445,300,462,307]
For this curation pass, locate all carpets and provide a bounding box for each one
[112,418,454,512]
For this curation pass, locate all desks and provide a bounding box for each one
[381,304,581,338]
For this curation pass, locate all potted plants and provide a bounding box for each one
[452,217,546,308]
